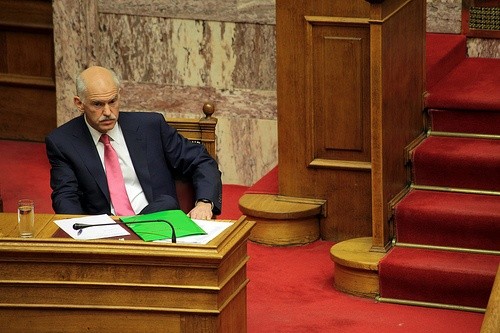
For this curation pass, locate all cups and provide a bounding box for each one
[17,199,35,238]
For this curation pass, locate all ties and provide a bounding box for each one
[99,134,136,216]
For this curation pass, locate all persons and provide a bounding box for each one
[45,66,223,220]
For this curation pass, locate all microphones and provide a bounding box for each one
[73,220,176,243]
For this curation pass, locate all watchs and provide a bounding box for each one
[195,199,213,209]
